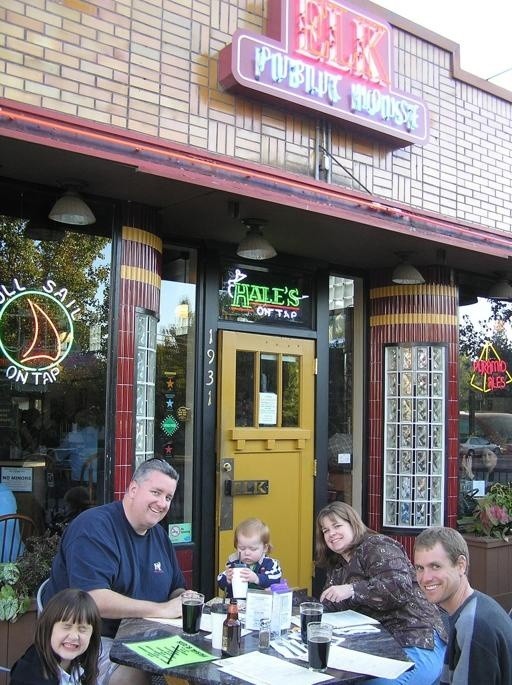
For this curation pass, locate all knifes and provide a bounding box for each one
[279,636,307,654]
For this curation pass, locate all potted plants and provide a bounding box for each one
[0,523,70,685]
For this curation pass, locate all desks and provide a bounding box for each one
[110,598,414,685]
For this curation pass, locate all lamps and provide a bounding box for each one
[392,251,426,285]
[236,218,277,260]
[48,183,96,225]
[488,271,512,299]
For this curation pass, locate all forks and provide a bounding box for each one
[275,639,300,658]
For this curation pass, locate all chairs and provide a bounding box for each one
[0,448,105,563]
[36,577,52,620]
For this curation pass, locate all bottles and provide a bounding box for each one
[221,599,242,657]
[258,619,271,649]
[271,578,289,592]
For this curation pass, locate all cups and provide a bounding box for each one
[181,593,205,637]
[229,564,249,599]
[307,622,332,674]
[210,602,229,650]
[300,601,323,645]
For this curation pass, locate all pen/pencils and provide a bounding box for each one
[167,644,180,664]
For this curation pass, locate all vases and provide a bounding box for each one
[456,482,512,542]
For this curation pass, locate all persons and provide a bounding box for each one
[216,517,282,599]
[0,467,28,563]
[413,525,512,685]
[328,420,353,478]
[8,403,107,500]
[460,445,506,487]
[8,588,103,685]
[309,501,449,685]
[42,458,200,685]
